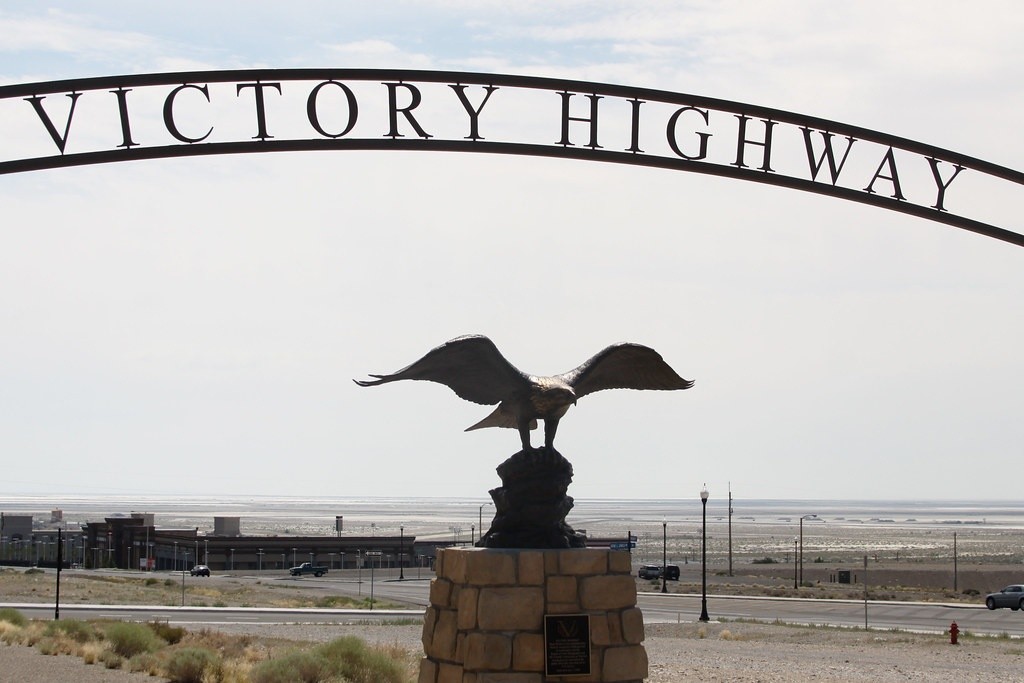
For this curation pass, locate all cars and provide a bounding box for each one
[985,584,1024,612]
[191,565,211,578]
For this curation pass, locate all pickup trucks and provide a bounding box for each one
[289,563,328,577]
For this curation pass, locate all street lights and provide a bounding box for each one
[427,556,432,568]
[480,502,492,539]
[328,553,336,570]
[310,552,314,567]
[356,556,360,572]
[661,514,669,594]
[698,483,711,622]
[471,522,476,546]
[1,531,264,574]
[292,548,298,567]
[420,554,426,569]
[800,514,817,587]
[340,552,346,571]
[399,521,405,579]
[386,555,391,580]
[281,554,285,572]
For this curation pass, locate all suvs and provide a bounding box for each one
[659,564,680,581]
[638,564,661,580]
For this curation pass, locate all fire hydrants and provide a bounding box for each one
[949,620,960,645]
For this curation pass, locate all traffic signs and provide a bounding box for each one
[365,549,383,557]
